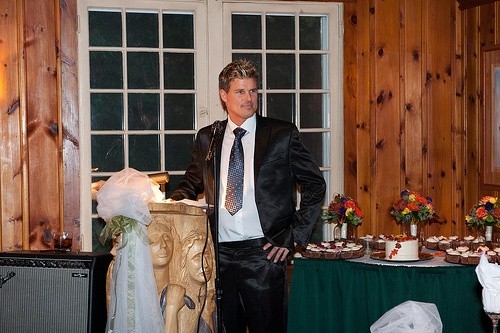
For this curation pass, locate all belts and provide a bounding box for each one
[220,238,269,248]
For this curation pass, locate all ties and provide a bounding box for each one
[225,128,247,216]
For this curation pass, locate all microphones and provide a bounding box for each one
[207,120,224,161]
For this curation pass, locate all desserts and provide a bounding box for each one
[357,234,387,250]
[301,239,364,259]
[426,235,500,264]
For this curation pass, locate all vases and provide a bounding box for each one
[410,222,417,238]
[340,222,348,240]
[485,225,492,242]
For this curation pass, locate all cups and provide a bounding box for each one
[57,231,72,252]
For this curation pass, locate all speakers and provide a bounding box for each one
[0,250,114,333]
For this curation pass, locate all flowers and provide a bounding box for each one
[320,193,365,228]
[388,189,436,224]
[465,195,500,228]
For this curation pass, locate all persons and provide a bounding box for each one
[165,59,326,333]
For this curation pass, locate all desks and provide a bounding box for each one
[286,245,500,333]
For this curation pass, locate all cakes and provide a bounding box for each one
[384,235,419,260]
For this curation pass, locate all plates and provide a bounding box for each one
[370,250,434,262]
